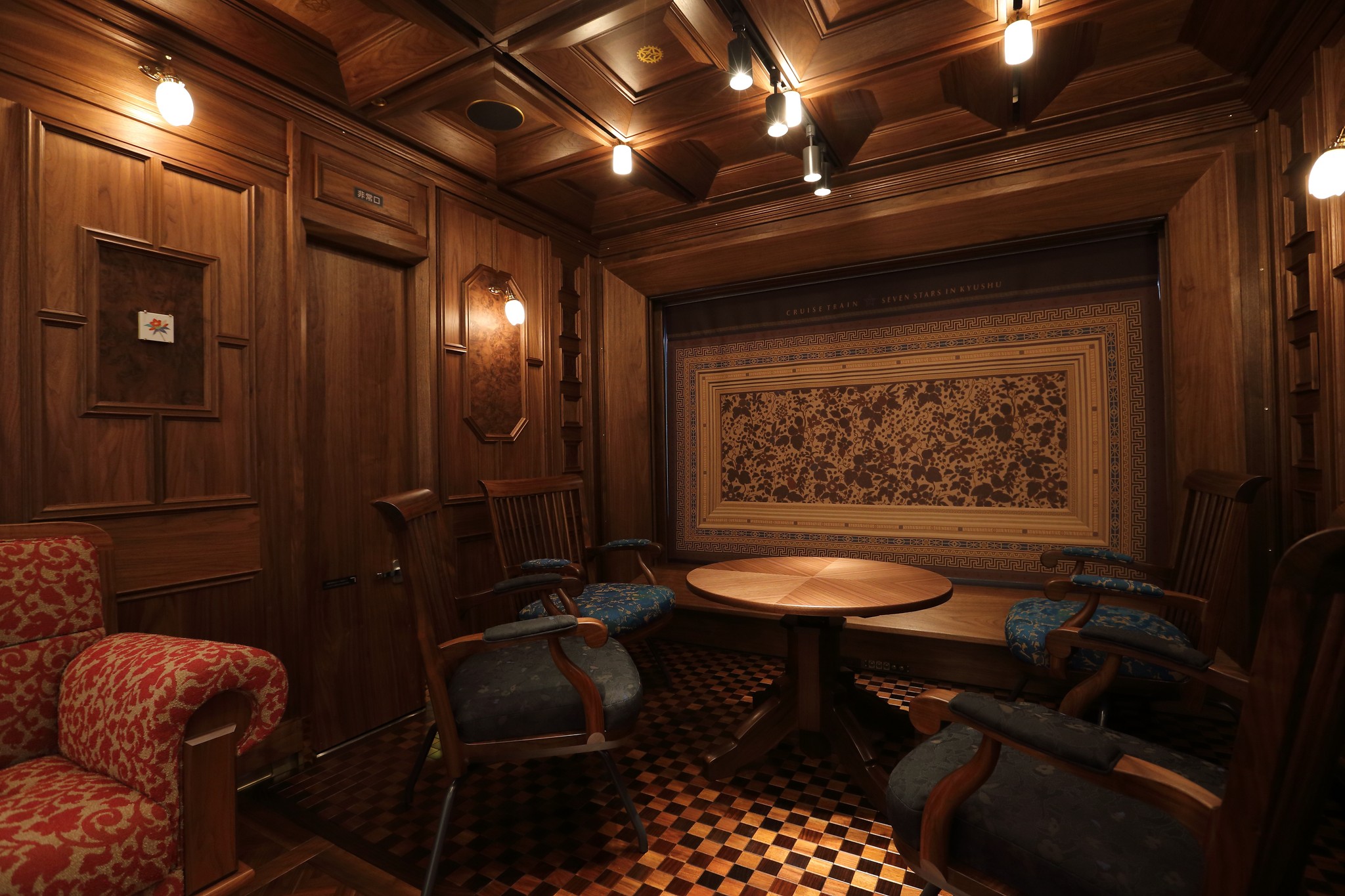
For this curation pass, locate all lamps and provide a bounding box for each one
[998,0,1037,69]
[610,142,635,177]
[802,123,821,182]
[814,147,835,197]
[766,69,790,138]
[1306,122,1345,201]
[726,16,754,91]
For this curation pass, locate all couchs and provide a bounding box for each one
[1,519,288,896]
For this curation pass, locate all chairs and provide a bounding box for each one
[999,468,1272,712]
[369,487,650,895]
[479,473,677,703]
[882,519,1343,896]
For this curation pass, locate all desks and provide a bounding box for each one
[683,555,957,806]
